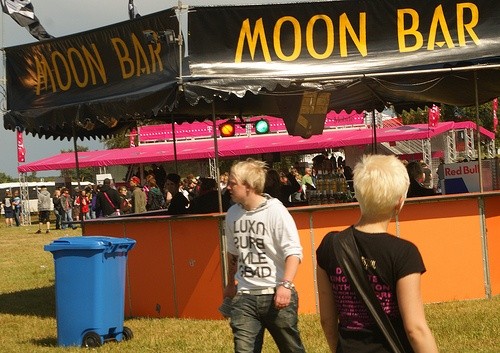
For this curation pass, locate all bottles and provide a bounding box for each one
[308,191,354,206]
[315,165,346,194]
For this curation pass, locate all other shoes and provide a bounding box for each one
[37,230,41,233]
[73,227,77,230]
[46,230,50,233]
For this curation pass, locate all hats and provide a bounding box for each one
[132,176,141,185]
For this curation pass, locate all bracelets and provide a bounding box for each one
[280,283,293,290]
[278,280,296,290]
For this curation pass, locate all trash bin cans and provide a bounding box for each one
[44,235,134,347]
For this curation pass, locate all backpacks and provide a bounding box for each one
[150,190,163,209]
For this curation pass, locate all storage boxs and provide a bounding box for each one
[443,159,492,192]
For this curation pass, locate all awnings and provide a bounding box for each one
[17,121,454,173]
[430,151,444,159]
[477,126,495,139]
[0,0,500,141]
[0,181,55,189]
[453,121,477,130]
[71,181,95,185]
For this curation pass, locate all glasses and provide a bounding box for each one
[128,180,133,182]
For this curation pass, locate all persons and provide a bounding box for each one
[3,191,21,227]
[223,160,308,353]
[315,154,440,353]
[405,156,443,195]
[35,186,51,233]
[53,163,237,230]
[265,151,354,206]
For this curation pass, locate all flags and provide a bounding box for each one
[428,103,440,130]
[493,97,498,132]
[129,130,136,148]
[17,128,25,162]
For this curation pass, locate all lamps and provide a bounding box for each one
[253,118,269,134]
[96,113,118,129]
[76,117,94,131]
[218,119,235,137]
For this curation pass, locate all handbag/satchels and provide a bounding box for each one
[115,209,120,216]
